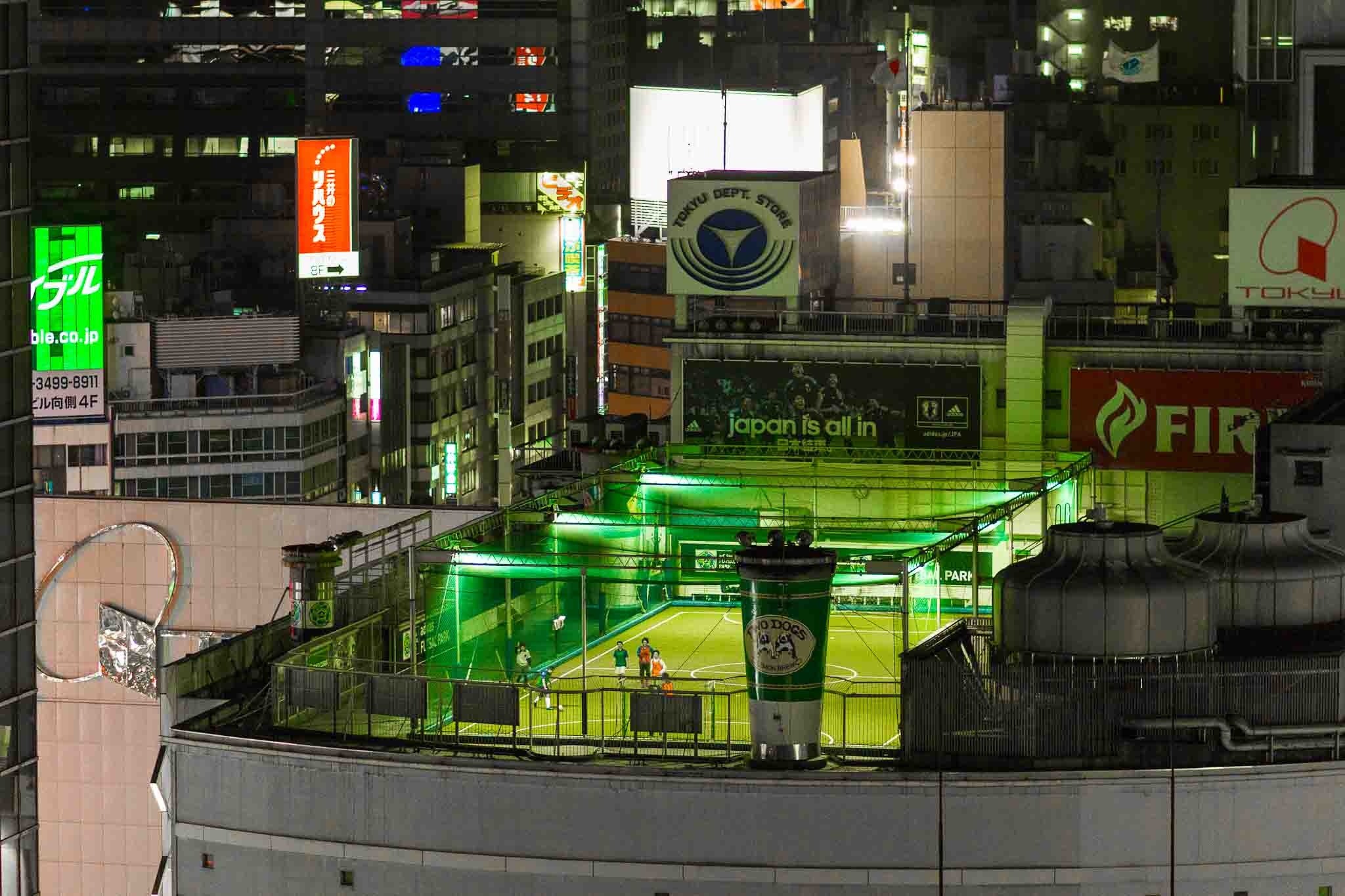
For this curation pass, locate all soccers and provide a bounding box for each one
[559,704,564,712]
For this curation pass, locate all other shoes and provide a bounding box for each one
[546,708,554,710]
[534,702,536,707]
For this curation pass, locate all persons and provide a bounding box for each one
[709,363,906,455]
[516,643,531,687]
[534,666,555,710]
[637,638,654,688]
[613,641,629,688]
[649,650,666,677]
[661,673,673,695]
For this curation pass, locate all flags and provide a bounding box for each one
[871,51,907,90]
[1102,40,1160,83]
